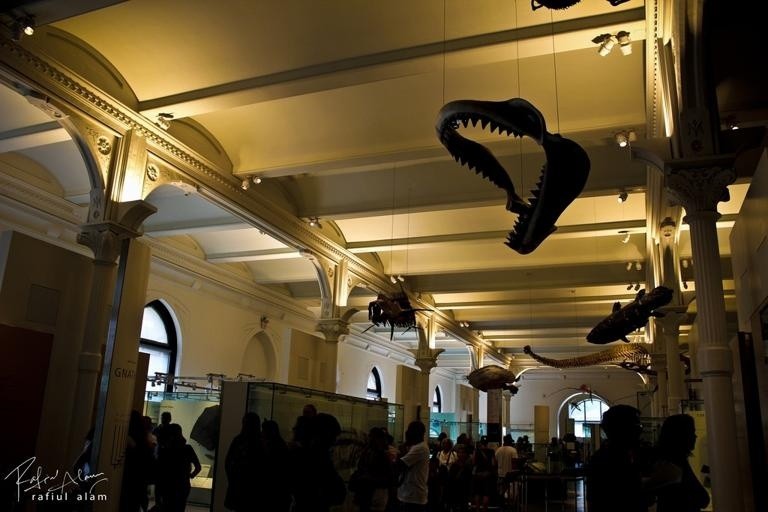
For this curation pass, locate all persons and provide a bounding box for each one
[651,413,711,511]
[120,402,582,511]
[581,405,675,511]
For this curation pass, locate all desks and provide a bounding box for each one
[521,473,584,511]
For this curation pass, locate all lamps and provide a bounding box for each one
[621,234,630,243]
[627,283,641,291]
[615,133,628,149]
[155,117,172,132]
[627,262,642,272]
[597,35,635,56]
[618,194,628,202]
[390,276,405,284]
[241,175,261,191]
